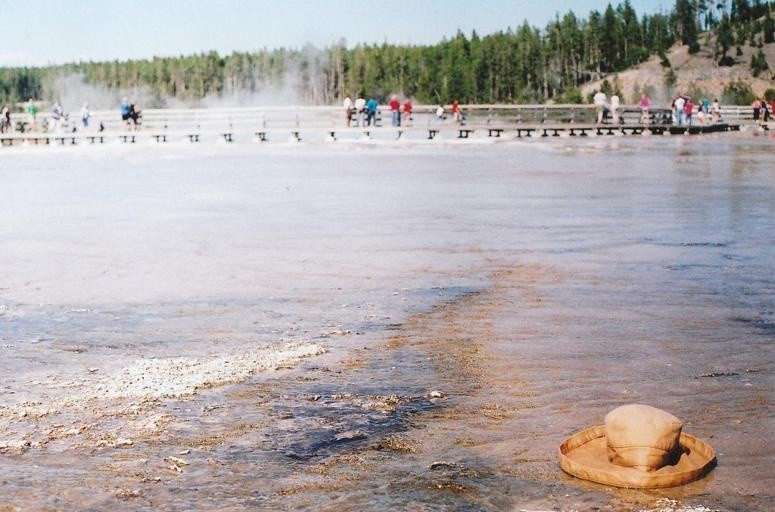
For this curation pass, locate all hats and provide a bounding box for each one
[557,404,718,489]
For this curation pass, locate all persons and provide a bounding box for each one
[761,95,768,122]
[81,103,91,128]
[592,89,609,124]
[638,93,652,113]
[388,96,400,126]
[26,98,37,121]
[402,99,412,122]
[453,99,460,120]
[672,93,721,125]
[609,90,620,123]
[366,94,378,125]
[355,93,366,126]
[752,98,761,120]
[344,93,354,127]
[437,105,444,119]
[130,99,142,125]
[50,99,60,125]
[2,103,9,134]
[121,96,130,126]
[772,97,775,114]
